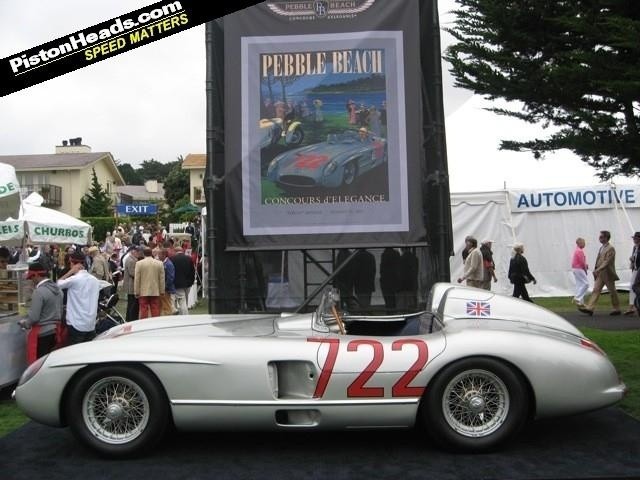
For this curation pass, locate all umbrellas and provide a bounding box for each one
[0,192,95,264]
[0,162,27,223]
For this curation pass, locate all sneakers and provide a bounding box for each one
[610,310,619,315]
[578,307,593,316]
[624,309,634,314]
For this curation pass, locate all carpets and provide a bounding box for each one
[1,406,639,480]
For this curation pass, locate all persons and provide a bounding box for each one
[352,248,377,305]
[576,231,627,315]
[380,248,400,307]
[456,239,483,289]
[264,97,324,123]
[479,239,497,291]
[1,209,201,362]
[624,231,640,316]
[462,236,474,264]
[347,96,387,135]
[393,247,419,309]
[334,249,355,306]
[570,236,591,306]
[508,242,537,303]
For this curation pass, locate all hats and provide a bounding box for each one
[25,263,46,279]
[483,240,494,244]
[632,232,640,240]
[67,253,84,263]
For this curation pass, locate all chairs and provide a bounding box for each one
[398,313,433,335]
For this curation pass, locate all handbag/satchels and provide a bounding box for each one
[521,273,531,283]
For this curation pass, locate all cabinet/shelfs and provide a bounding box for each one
[1,268,29,389]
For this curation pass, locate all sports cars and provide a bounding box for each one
[9,276,628,456]
[267,128,387,194]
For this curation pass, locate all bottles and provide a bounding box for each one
[0,269,8,280]
[18,303,28,317]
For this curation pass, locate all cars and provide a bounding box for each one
[259,119,304,146]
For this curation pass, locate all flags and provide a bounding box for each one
[466,302,491,317]
